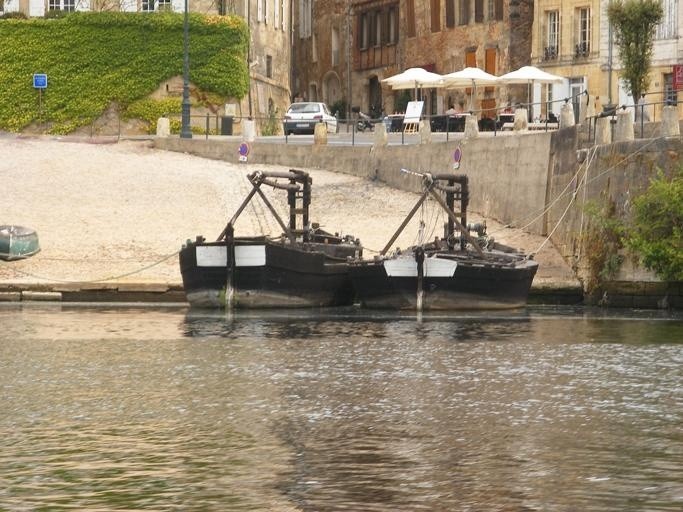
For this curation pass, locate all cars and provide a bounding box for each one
[280,102,338,135]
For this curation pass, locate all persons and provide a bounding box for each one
[431,236,442,249]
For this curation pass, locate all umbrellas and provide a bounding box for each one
[380,64,563,122]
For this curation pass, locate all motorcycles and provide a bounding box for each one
[352,105,386,132]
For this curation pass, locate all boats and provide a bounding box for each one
[0,227,40,262]
[181,170,393,293]
[377,171,540,295]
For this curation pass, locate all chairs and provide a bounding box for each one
[387,109,558,132]
